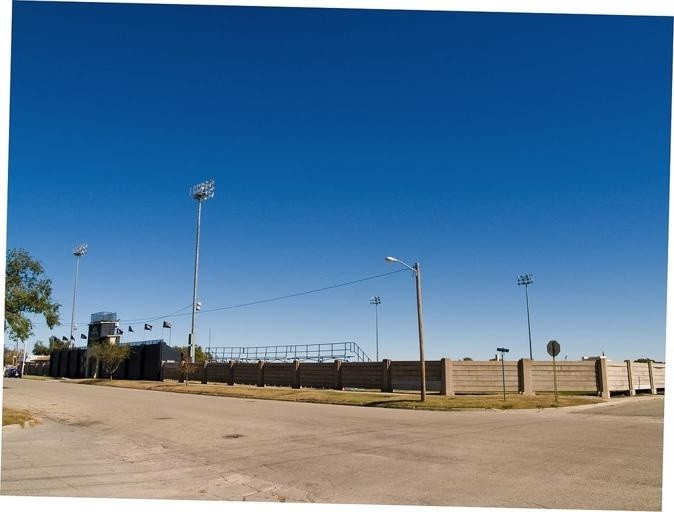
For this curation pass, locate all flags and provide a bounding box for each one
[144,323,152,331]
[52,336,60,342]
[70,335,75,341]
[116,328,124,335]
[62,336,68,341]
[128,325,134,332]
[162,320,173,329]
[81,334,87,339]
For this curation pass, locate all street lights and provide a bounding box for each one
[69,240,89,347]
[517,273,535,360]
[12,355,16,365]
[369,295,382,362]
[189,178,216,364]
[385,256,427,403]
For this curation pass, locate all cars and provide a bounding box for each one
[3,368,9,377]
[8,368,21,378]
